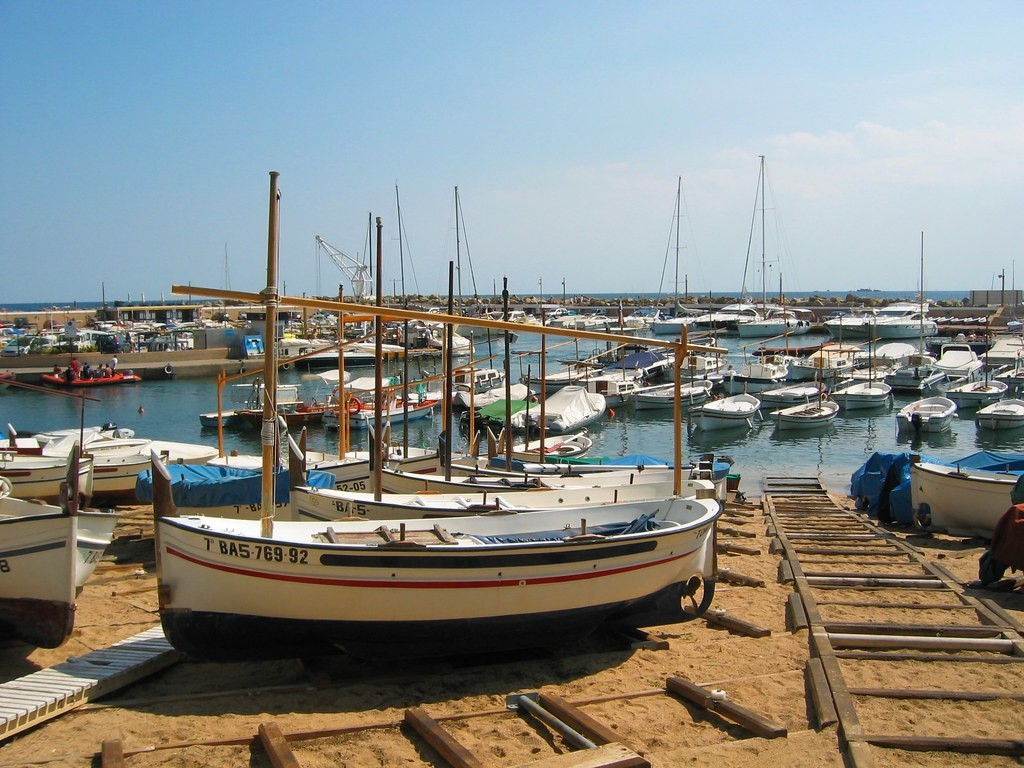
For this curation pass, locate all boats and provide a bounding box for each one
[0,395,743,649]
[927,315,987,325]
[790,356,856,380]
[908,454,1021,536]
[509,384,608,433]
[688,393,760,432]
[920,342,984,394]
[721,342,786,394]
[976,398,1024,429]
[289,347,383,370]
[0,370,16,389]
[41,370,142,386]
[991,356,1024,390]
[896,395,958,433]
[623,380,714,412]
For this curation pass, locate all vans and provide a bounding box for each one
[502,311,527,324]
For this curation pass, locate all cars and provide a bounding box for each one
[562,310,581,316]
[0,307,186,355]
[544,308,567,317]
[584,309,610,316]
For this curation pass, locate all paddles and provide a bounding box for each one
[545,431,587,453]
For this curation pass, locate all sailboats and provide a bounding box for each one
[190,152,938,434]
[691,290,726,386]
[945,316,1009,409]
[831,313,890,410]
[836,315,889,382]
[769,342,839,430]
[884,231,938,391]
[758,295,802,381]
[1007,260,1024,331]
[148,172,728,664]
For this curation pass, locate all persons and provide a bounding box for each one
[397,324,402,346]
[95,363,107,378]
[429,325,432,335]
[113,335,124,354]
[110,355,120,376]
[54,364,63,376]
[66,365,74,381]
[83,363,94,378]
[138,406,144,414]
[159,326,166,335]
[126,332,136,354]
[978,473,1024,584]
[101,364,112,378]
[956,330,997,343]
[70,357,81,378]
[311,329,318,338]
[334,330,339,340]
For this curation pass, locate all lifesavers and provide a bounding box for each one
[345,397,361,414]
[164,365,173,375]
[240,367,247,374]
[283,363,290,369]
